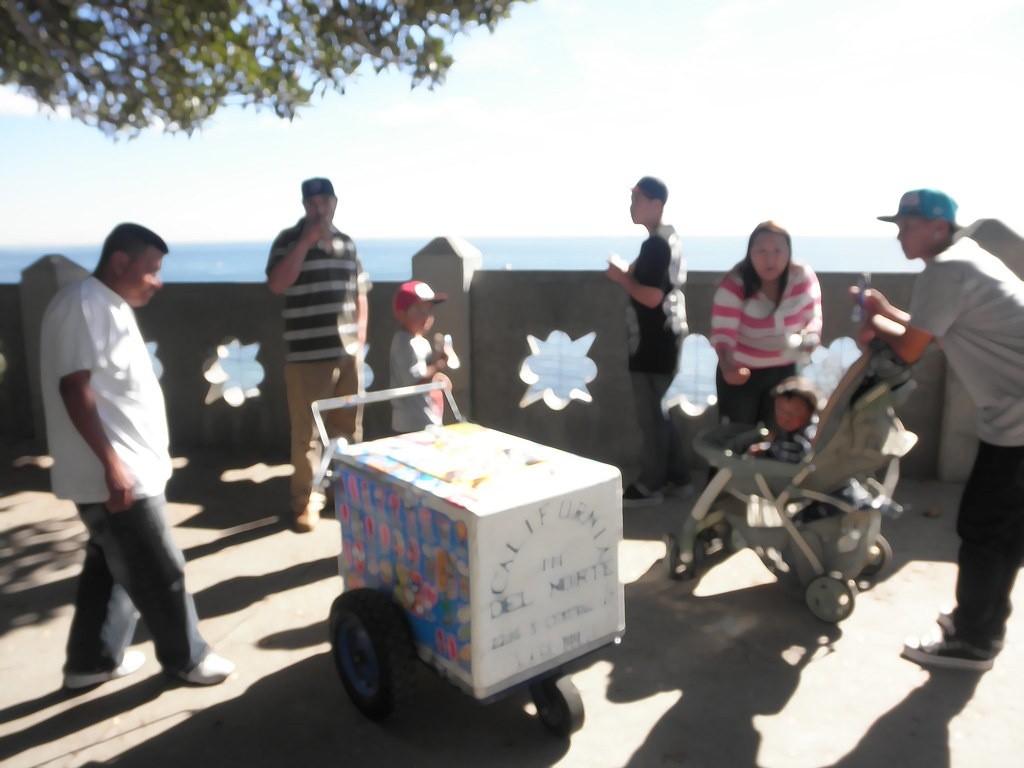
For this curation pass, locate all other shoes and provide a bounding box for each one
[293,504,321,531]
[901,640,995,670]
[937,611,1006,649]
[63,652,146,689]
[169,651,234,685]
[623,474,694,506]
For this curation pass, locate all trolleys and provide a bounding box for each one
[310,382,621,738]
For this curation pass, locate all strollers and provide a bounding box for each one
[666,338,918,624]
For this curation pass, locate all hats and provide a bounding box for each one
[302,179,335,199]
[878,189,956,225]
[393,281,447,315]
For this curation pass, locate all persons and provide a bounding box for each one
[606,176,688,498]
[748,378,819,464]
[389,280,449,436]
[849,189,1024,670]
[42,223,234,688]
[265,177,368,530]
[700,222,823,541]
